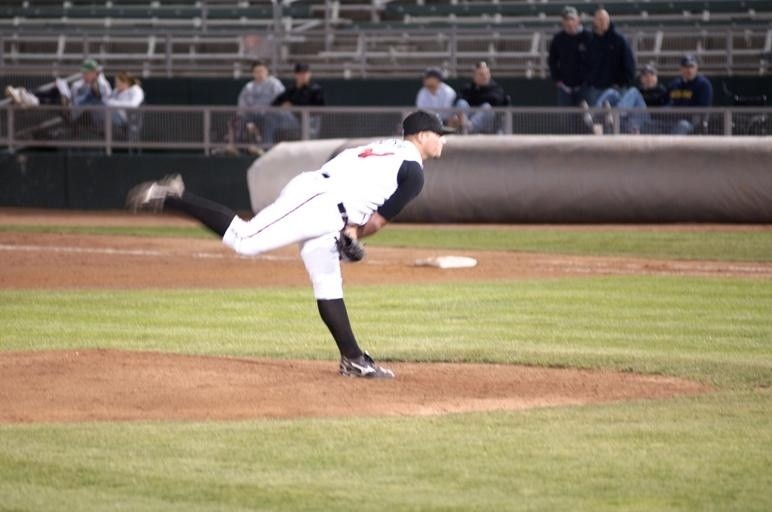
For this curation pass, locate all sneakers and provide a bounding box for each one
[126,173,185,217]
[6,85,39,109]
[337,350,394,380]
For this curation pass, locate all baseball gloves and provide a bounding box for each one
[339,232,363,263]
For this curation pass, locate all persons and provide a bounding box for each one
[666,54,715,136]
[122,108,459,382]
[581,63,669,134]
[222,61,287,159]
[546,5,595,134]
[578,8,637,134]
[449,57,510,136]
[415,66,458,137]
[264,61,326,150]
[4,57,113,112]
[43,69,145,145]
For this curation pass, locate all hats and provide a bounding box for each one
[640,64,657,74]
[418,68,442,79]
[476,60,489,69]
[561,6,577,19]
[403,110,458,136]
[681,55,697,67]
[294,63,309,72]
[80,59,99,72]
